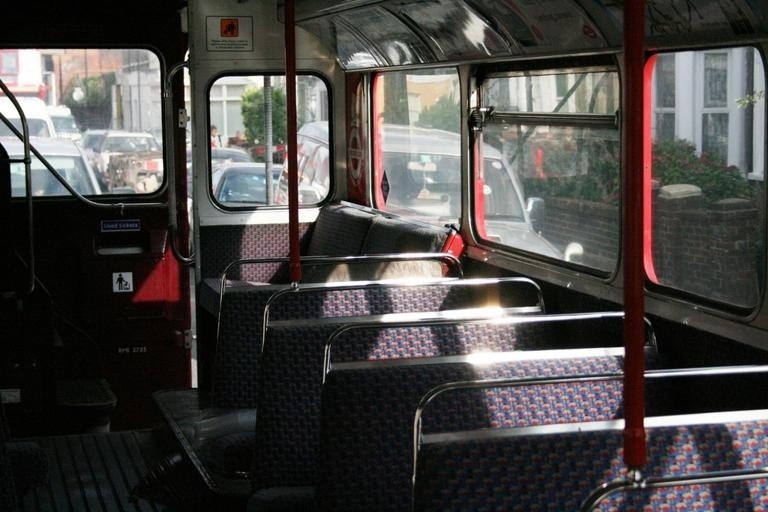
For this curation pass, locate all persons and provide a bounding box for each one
[209,125,225,149]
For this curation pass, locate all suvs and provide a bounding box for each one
[276,116,583,268]
[0,93,100,196]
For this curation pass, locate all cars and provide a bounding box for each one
[87,127,281,207]
